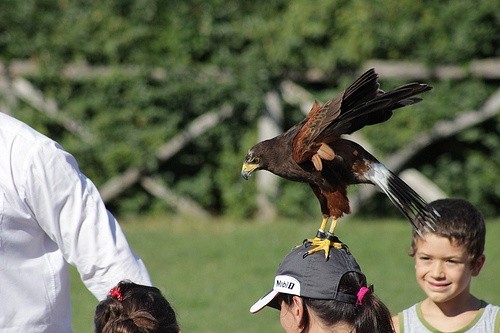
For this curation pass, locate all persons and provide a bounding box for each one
[0,112,152,333]
[390,199,500,333]
[249,239,396,333]
[93,281,182,333]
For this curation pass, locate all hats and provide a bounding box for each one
[249,237,375,313]
